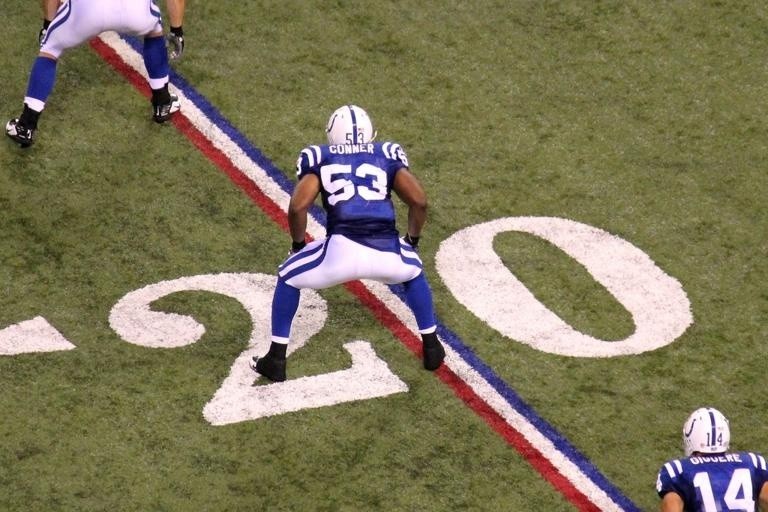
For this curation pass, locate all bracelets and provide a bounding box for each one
[43,18,52,30]
[169,25,183,37]
[292,237,306,253]
[404,231,420,248]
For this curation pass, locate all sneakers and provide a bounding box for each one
[256,352,286,381]
[152,92,181,123]
[423,341,445,371]
[5,117,34,146]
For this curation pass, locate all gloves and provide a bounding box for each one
[39,18,51,45]
[165,26,184,60]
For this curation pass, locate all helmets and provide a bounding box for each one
[682,407,730,457]
[325,105,373,145]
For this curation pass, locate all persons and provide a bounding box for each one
[249,102,446,383]
[656,405,768,512]
[5,0,187,145]
